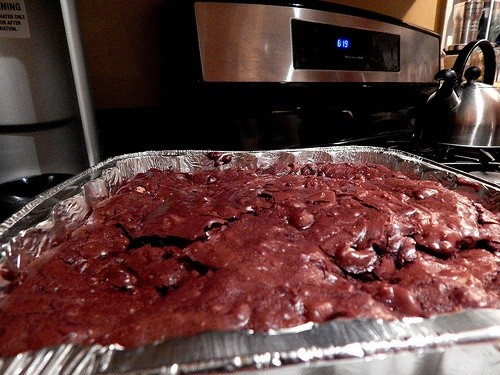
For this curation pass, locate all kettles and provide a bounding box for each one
[417,38,500,157]
